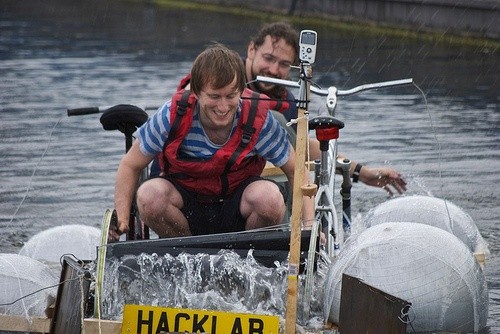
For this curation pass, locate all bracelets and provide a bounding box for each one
[352,163,363,183]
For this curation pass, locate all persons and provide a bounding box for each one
[177,22,407,196]
[107,41,327,247]
